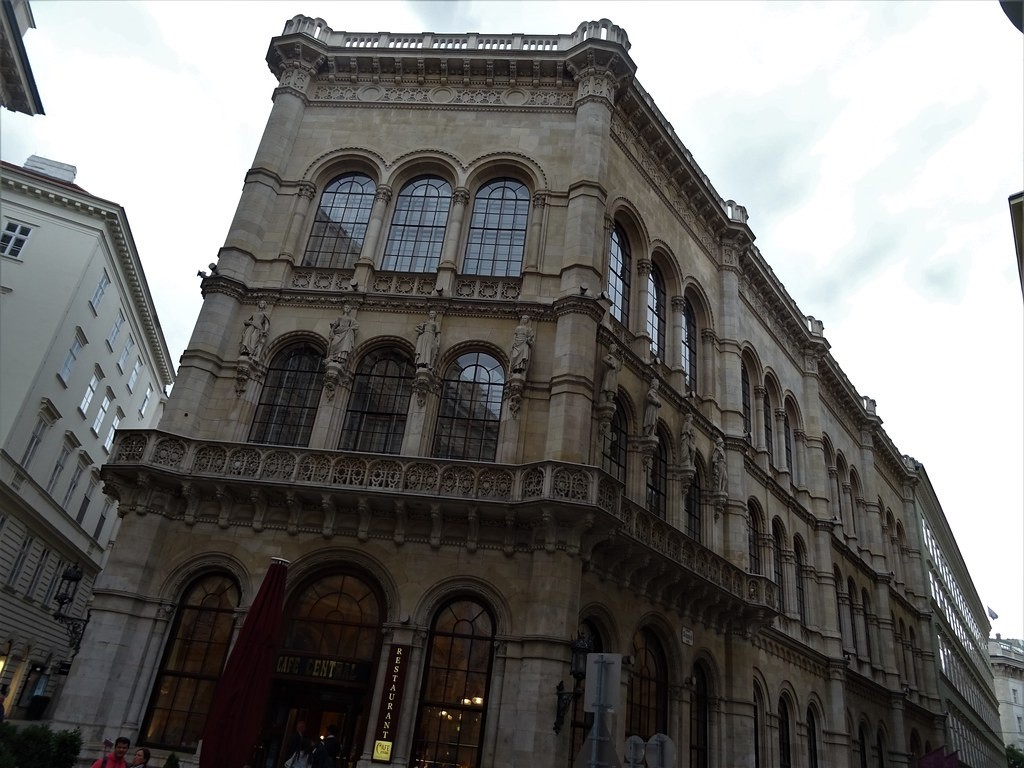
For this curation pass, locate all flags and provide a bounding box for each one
[918,746,945,768]
[945,751,960,768]
[988,607,999,620]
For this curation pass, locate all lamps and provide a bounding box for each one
[400,612,410,624]
[552,625,594,736]
[50,554,92,657]
[682,675,698,688]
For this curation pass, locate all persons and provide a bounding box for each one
[681,414,694,467]
[129,749,150,768]
[603,343,625,404]
[328,304,359,363]
[415,310,441,370]
[643,379,662,438]
[509,315,535,374]
[711,437,728,493]
[238,300,271,357]
[285,721,341,768]
[92,736,130,768]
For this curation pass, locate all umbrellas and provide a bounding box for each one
[198,556,291,768]
